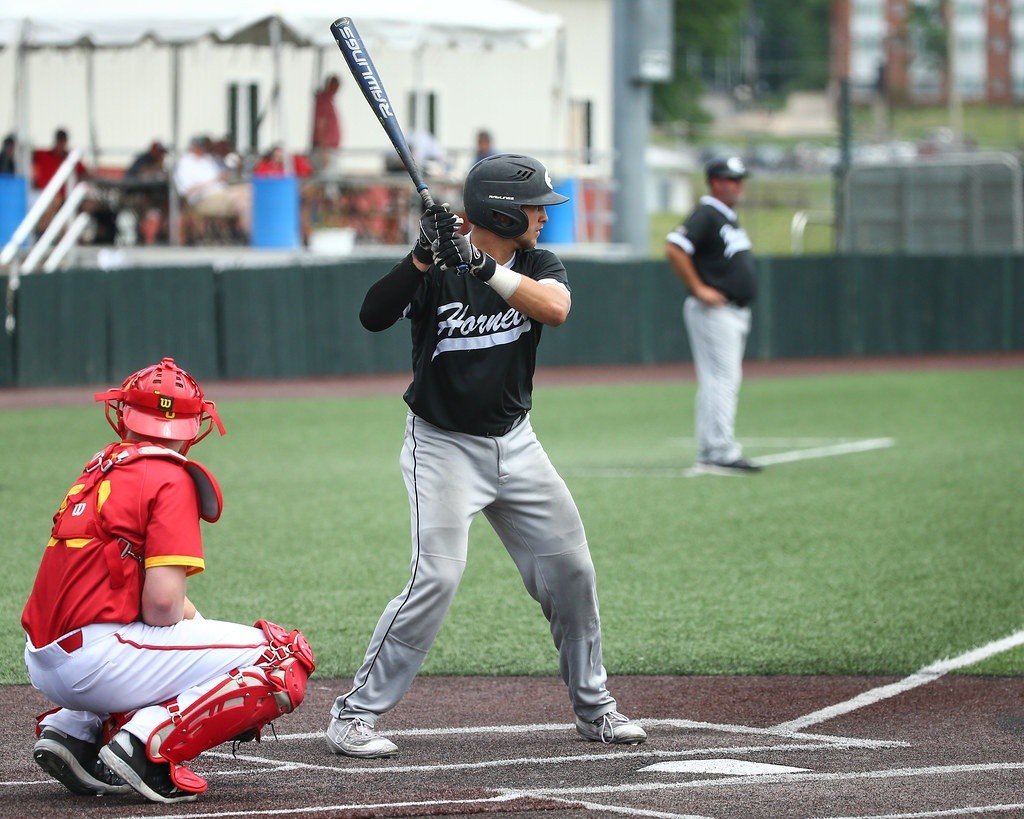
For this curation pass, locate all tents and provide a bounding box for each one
[0,1,568,246]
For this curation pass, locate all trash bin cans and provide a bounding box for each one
[539,178,580,242]
[0,173,33,248]
[249,176,306,248]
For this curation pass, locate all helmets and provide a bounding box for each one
[123,364,201,439]
[462,154,570,239]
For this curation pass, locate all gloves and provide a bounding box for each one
[431,233,496,282]
[412,203,464,265]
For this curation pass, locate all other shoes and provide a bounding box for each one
[716,453,759,477]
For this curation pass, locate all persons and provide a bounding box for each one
[22,357,314,800]
[1,77,494,246]
[324,154,648,759]
[664,155,760,472]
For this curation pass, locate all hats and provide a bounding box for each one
[706,155,751,180]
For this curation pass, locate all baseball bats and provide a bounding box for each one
[330,17,470,277]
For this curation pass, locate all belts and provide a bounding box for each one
[57,629,82,653]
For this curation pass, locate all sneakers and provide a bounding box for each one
[575,709,648,745]
[326,715,398,758]
[33,725,132,795]
[97,728,197,803]
